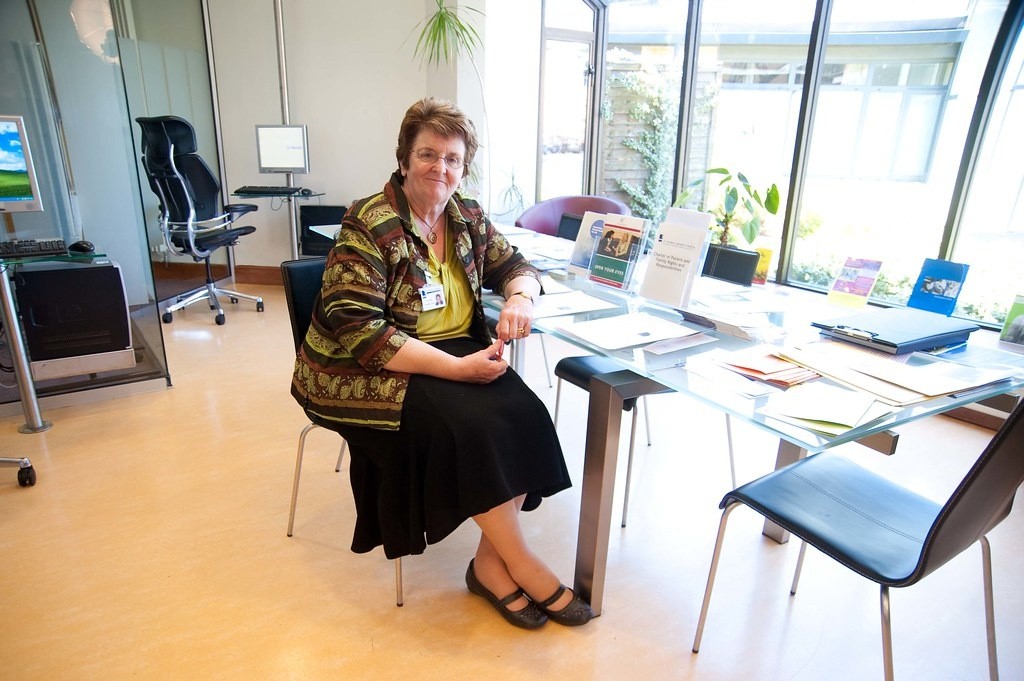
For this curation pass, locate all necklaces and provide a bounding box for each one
[409,203,443,244]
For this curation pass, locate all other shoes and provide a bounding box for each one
[523,581,592,626]
[465,557,548,629]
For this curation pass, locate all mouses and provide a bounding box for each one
[69,240,95,252]
[301,189,311,194]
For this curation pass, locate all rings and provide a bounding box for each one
[517,328,524,333]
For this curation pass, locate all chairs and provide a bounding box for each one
[299,205,348,259]
[131,113,1024,681]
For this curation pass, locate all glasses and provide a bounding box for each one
[408,147,469,170]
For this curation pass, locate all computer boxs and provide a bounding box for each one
[14,258,133,361]
[300,205,348,258]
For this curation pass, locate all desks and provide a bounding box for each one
[308,221,1024,619]
[0,251,107,434]
[230,191,326,260]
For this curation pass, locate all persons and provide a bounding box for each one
[291,98,593,628]
[435,294,443,305]
[597,231,631,259]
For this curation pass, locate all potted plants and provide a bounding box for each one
[672,168,780,287]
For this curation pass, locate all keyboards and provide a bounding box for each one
[0,238,68,260]
[234,185,302,195]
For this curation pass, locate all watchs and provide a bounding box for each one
[512,290,535,305]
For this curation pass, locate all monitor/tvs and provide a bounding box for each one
[0,113,44,213]
[256,124,312,175]
